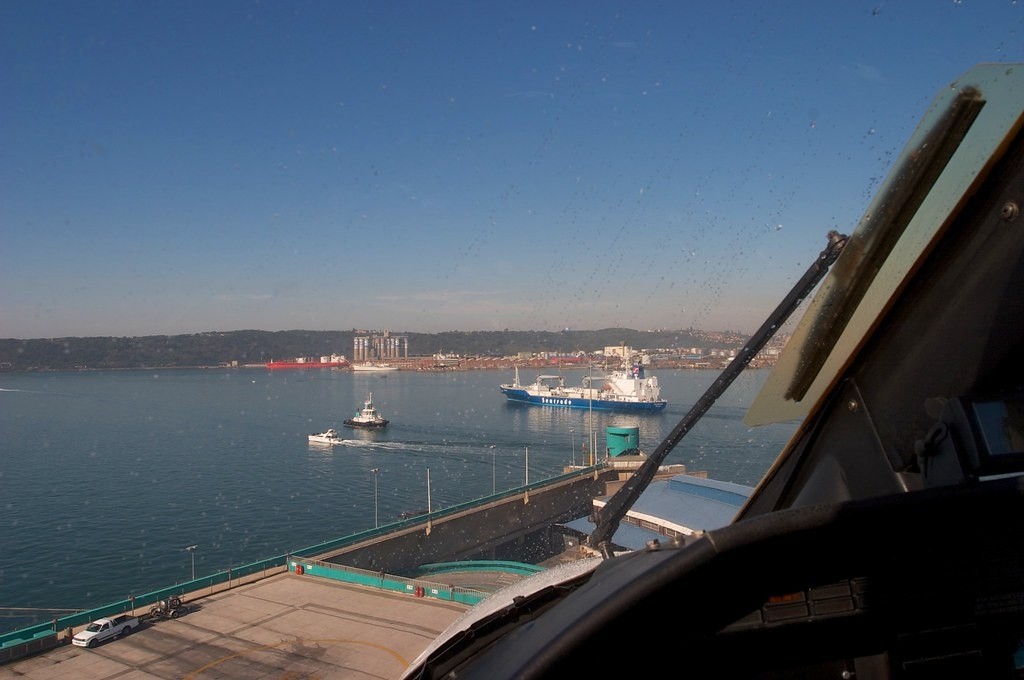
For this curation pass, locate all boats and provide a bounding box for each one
[343,392,390,428]
[353,362,399,371]
[309,434,343,444]
[500,345,667,410]
